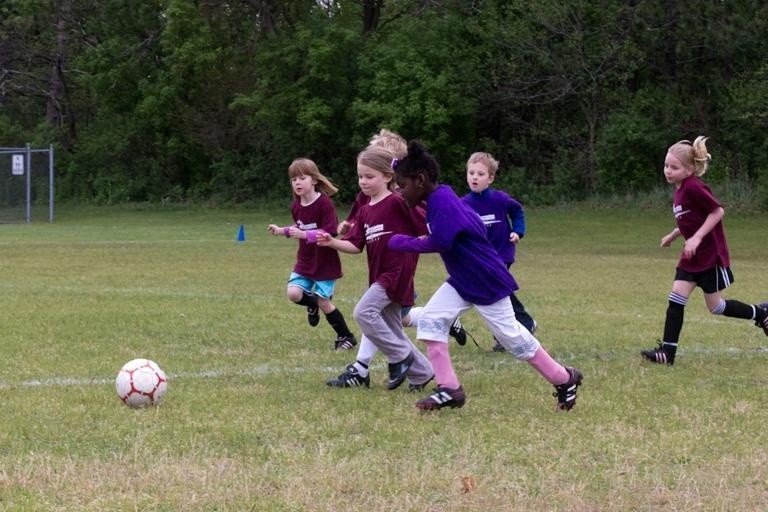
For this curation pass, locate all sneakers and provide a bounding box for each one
[529,318,538,336]
[641,347,676,368]
[407,368,434,392]
[552,368,583,414]
[327,364,371,389]
[448,316,481,352]
[333,337,359,352]
[306,292,321,327]
[758,303,768,336]
[386,350,416,391]
[494,344,507,355]
[415,385,468,411]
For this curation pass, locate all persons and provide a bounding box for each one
[326,128,468,394]
[459,152,538,353]
[268,158,357,351]
[391,141,584,414]
[641,136,768,367]
[317,147,436,393]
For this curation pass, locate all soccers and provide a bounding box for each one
[116,358,168,409]
